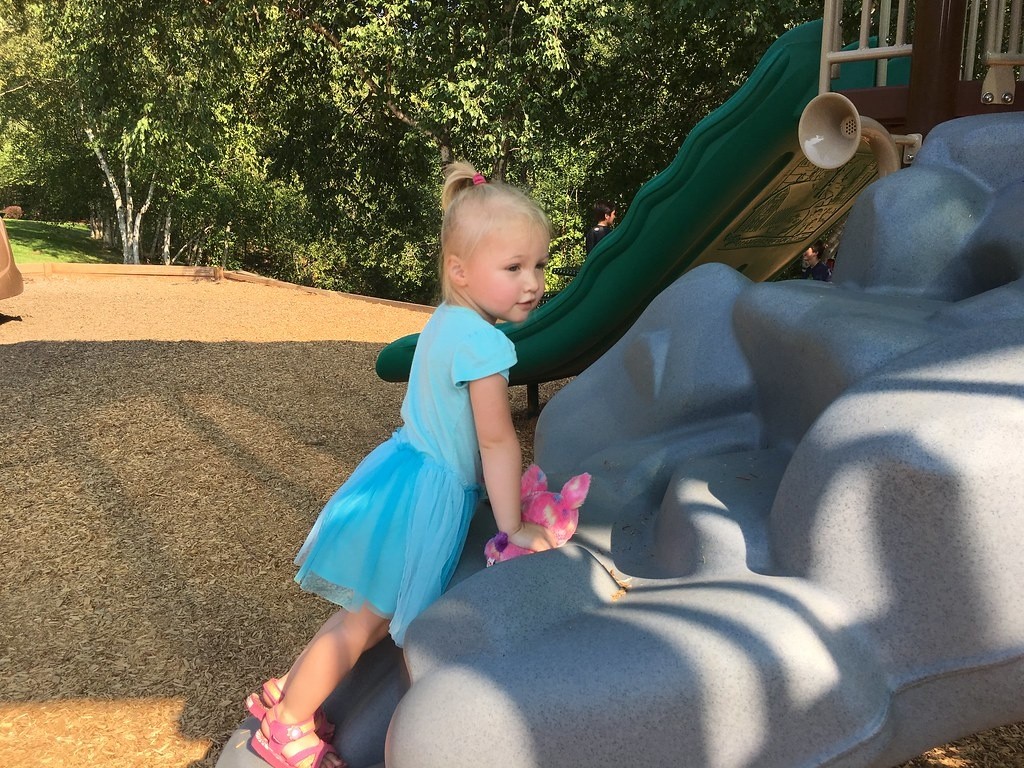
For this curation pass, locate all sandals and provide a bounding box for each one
[244,676,336,735]
[251,700,344,768]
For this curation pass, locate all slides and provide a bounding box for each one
[374,17,912,389]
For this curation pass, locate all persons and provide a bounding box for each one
[586,200,617,258]
[244,159,553,768]
[797,239,831,282]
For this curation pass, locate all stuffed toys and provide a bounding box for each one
[484,465,591,569]
